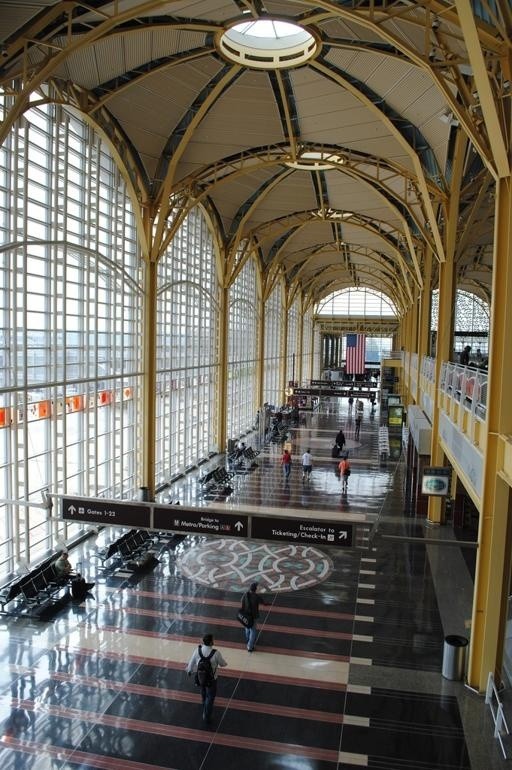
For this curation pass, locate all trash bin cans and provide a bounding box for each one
[442,635,468,680]
[228,440,234,452]
[139,488,147,502]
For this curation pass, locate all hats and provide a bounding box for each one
[250,582,259,590]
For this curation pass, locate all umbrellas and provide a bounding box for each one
[71,580,87,602]
[83,582,95,589]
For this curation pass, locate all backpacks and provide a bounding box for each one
[195,645,217,688]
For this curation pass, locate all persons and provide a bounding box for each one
[354,417,361,437]
[475,347,482,362]
[280,450,293,479]
[340,458,348,492]
[241,580,271,652]
[254,408,259,429]
[301,450,314,482]
[335,428,345,452]
[461,344,472,366]
[186,633,228,724]
[347,387,354,404]
[56,552,72,582]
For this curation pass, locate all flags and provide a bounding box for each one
[344,333,366,376]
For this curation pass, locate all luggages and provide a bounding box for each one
[72,602,86,622]
[72,561,87,602]
[331,444,339,458]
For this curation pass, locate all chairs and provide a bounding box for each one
[0,549,82,616]
[94,528,161,570]
[197,446,260,492]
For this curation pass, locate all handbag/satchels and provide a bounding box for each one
[236,608,254,630]
[343,469,350,476]
